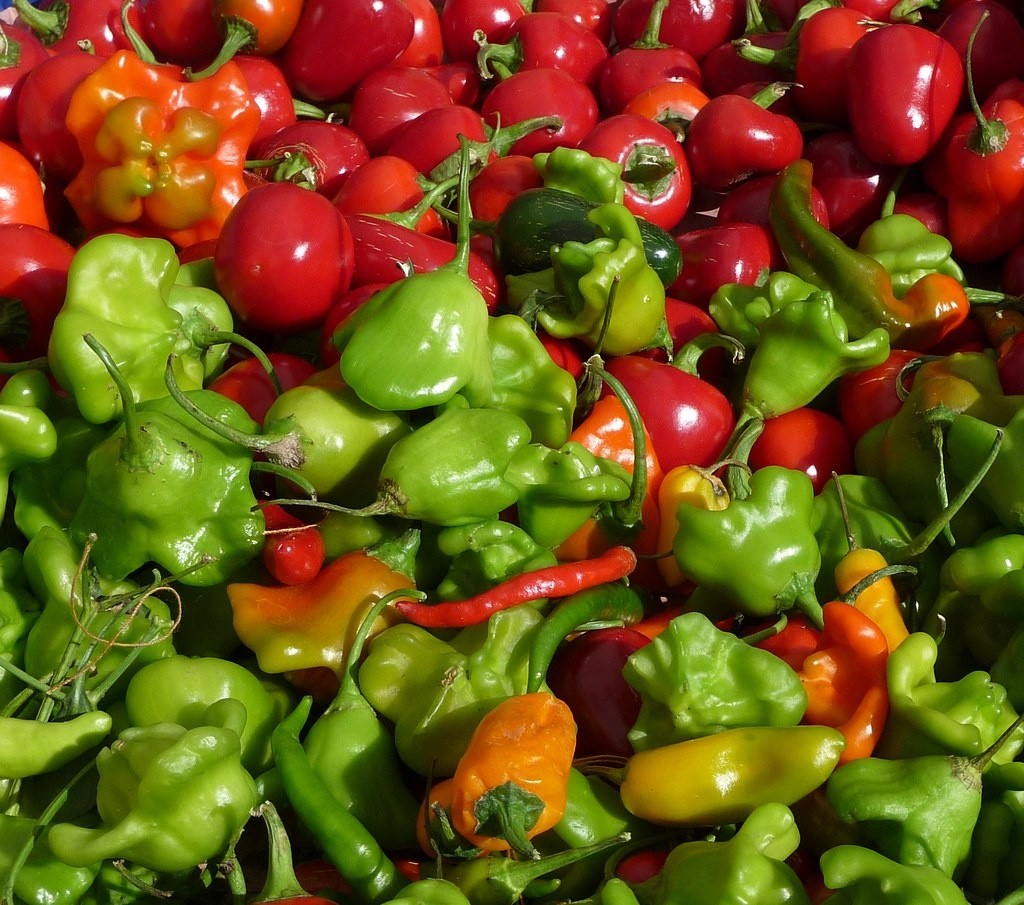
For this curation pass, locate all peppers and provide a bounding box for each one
[0,0,1024,905]
